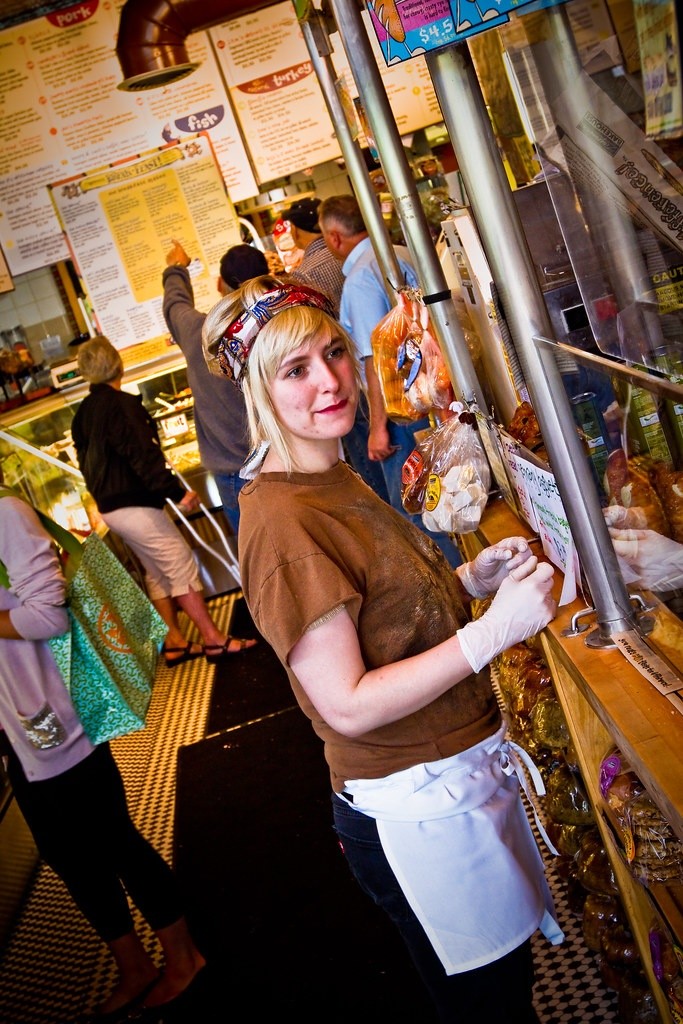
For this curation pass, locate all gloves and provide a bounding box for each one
[609,527,683,592]
[456,536,534,600]
[455,554,557,674]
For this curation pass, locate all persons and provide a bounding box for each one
[202,274,564,1024]
[71,338,259,668]
[0,485,219,1024]
[604,503,683,592]
[163,196,473,625]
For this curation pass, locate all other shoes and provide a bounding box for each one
[143,949,207,1012]
[100,961,159,1017]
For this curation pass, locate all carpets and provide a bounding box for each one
[165,597,440,1024]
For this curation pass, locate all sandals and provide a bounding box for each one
[204,634,258,663]
[163,640,205,669]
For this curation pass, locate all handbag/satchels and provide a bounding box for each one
[0,489,169,745]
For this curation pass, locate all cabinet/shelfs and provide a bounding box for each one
[412,427,683,1024]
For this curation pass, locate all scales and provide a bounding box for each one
[49,359,84,391]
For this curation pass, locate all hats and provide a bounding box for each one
[282,198,323,234]
[220,244,269,290]
